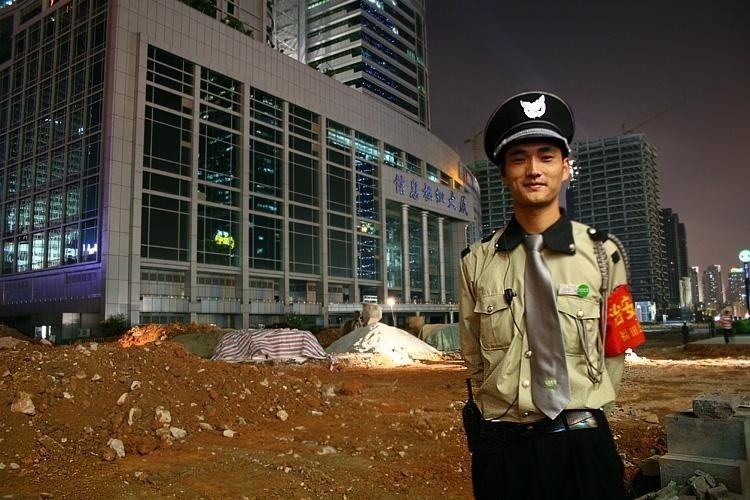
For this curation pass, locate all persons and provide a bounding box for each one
[456,89,651,498]
[681,322,689,345]
[719,310,734,345]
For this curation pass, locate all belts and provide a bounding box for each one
[487,410,599,437]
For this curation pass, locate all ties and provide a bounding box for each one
[523,233,571,421]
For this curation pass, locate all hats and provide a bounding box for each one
[482,91,576,164]
